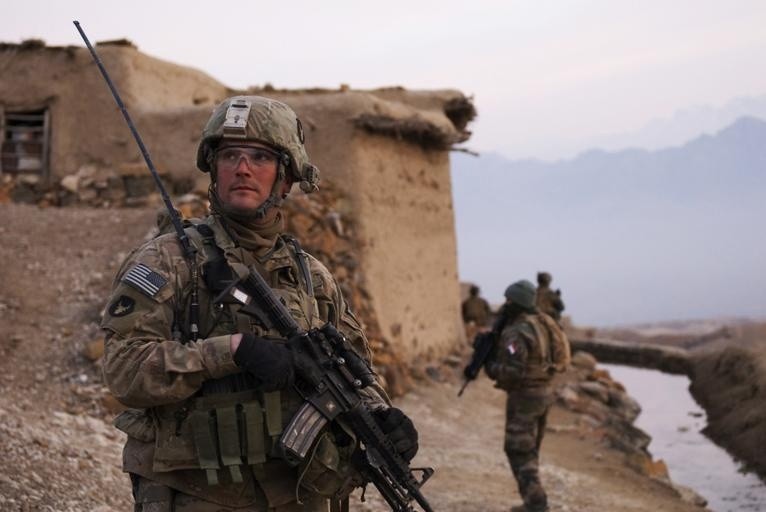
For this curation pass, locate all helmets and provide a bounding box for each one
[505,280,536,309]
[538,272,551,284]
[197,95,319,194]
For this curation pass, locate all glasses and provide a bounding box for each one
[220,145,281,168]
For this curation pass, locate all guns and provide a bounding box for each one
[211,268,439,512]
[457,300,527,396]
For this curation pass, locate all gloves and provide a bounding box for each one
[233,331,296,392]
[376,408,418,461]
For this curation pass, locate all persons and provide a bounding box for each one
[461,285,491,346]
[100,96,420,512]
[535,271,565,324]
[483,279,572,512]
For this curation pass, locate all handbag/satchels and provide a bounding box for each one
[298,433,349,499]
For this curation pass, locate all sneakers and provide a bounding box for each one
[512,504,548,512]
